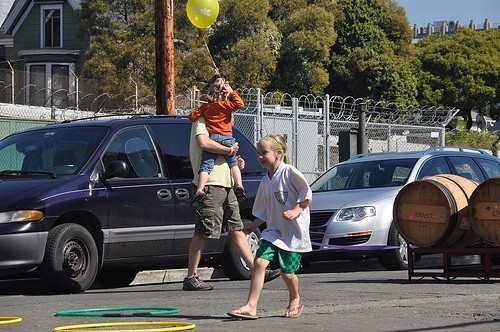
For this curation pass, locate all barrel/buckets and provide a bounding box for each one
[467,176,500,247]
[393,173,480,249]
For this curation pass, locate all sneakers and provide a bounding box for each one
[183,272,213,291]
[263,270,281,282]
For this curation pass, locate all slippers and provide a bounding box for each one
[226,309,259,319]
[285,303,304,317]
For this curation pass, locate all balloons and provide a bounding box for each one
[186,0,220,29]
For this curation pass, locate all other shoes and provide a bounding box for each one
[191,191,206,207]
[234,185,247,202]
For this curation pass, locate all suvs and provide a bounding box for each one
[0,112,281,295]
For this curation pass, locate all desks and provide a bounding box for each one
[407,244,500,283]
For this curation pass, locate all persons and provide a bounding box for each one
[225,132,313,320]
[187,75,246,207]
[181,79,281,291]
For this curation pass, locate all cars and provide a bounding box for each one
[301,146,500,270]
